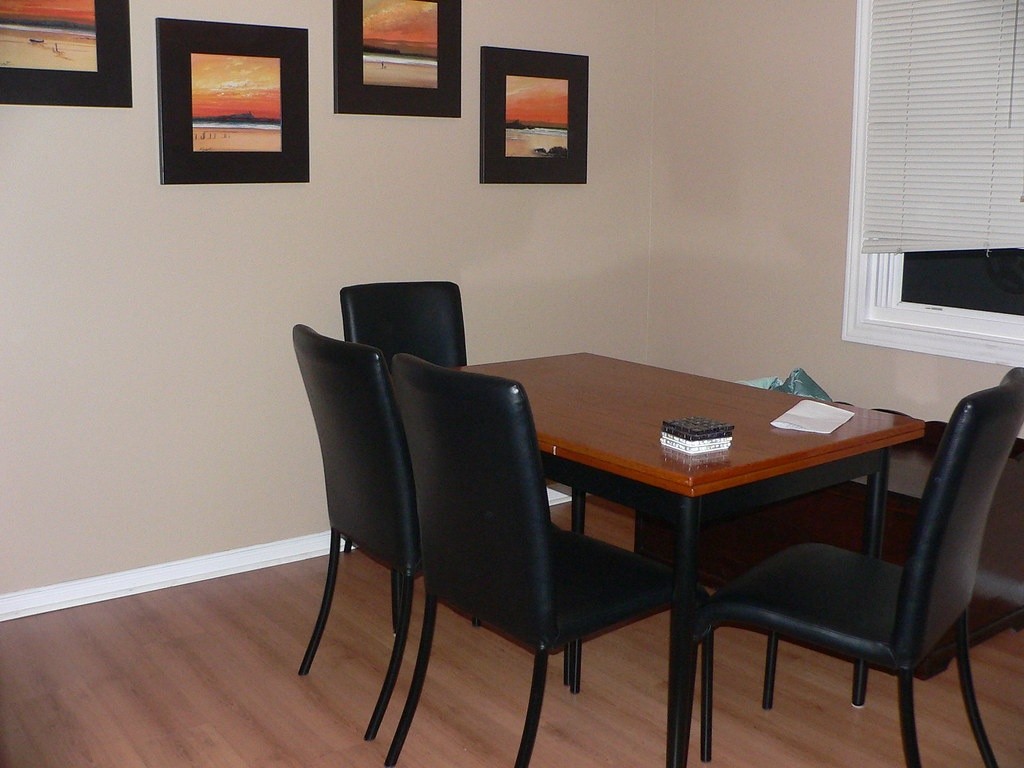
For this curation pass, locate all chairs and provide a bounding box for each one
[340,281,467,553]
[384,352,713,768]
[693,367,1023,768]
[293,324,422,743]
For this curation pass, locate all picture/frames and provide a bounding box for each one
[0,0,133,108]
[155,18,310,184]
[332,0,462,118]
[480,46,589,185]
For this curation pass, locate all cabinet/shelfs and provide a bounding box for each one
[634,401,1024,679]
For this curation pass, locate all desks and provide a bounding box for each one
[442,353,926,762]
